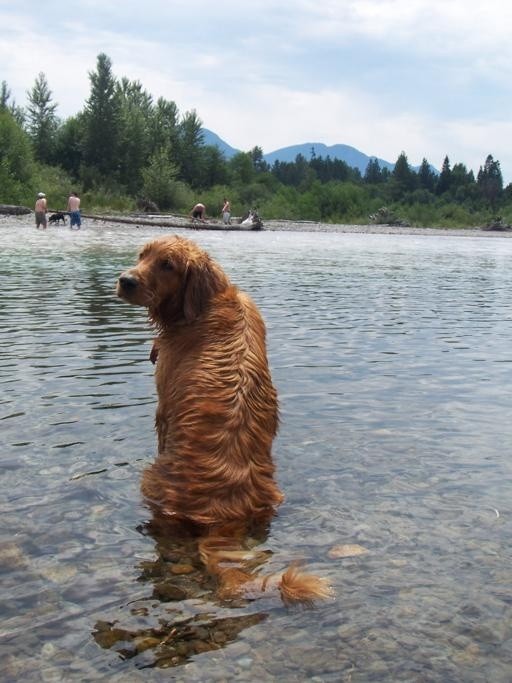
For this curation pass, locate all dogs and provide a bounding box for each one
[116,233,335,612]
[48,212,66,225]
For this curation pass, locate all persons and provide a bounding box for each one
[67,192,81,231]
[221,198,231,225]
[188,203,205,223]
[35,192,47,231]
